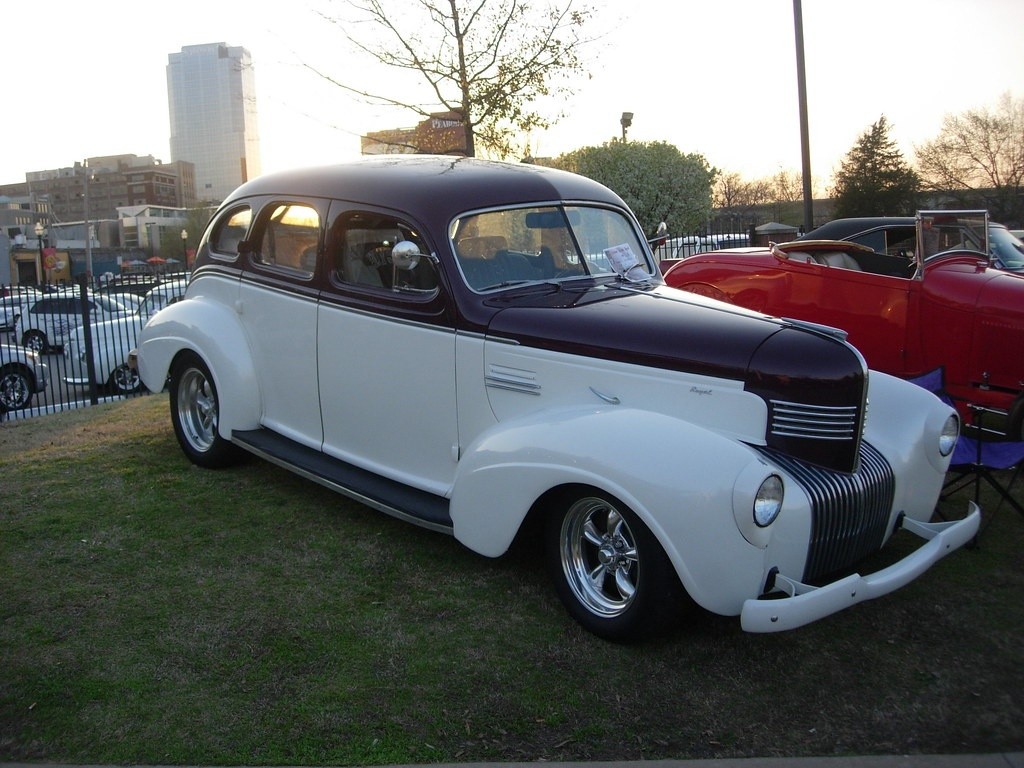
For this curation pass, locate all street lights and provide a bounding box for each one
[34,221,47,284]
[181,228,187,271]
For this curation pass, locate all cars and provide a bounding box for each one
[123,152,983,644]
[0,270,192,416]
[660,211,1024,459]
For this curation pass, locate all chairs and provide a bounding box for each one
[459,235,536,293]
[351,241,395,266]
[788,251,817,264]
[817,252,863,273]
